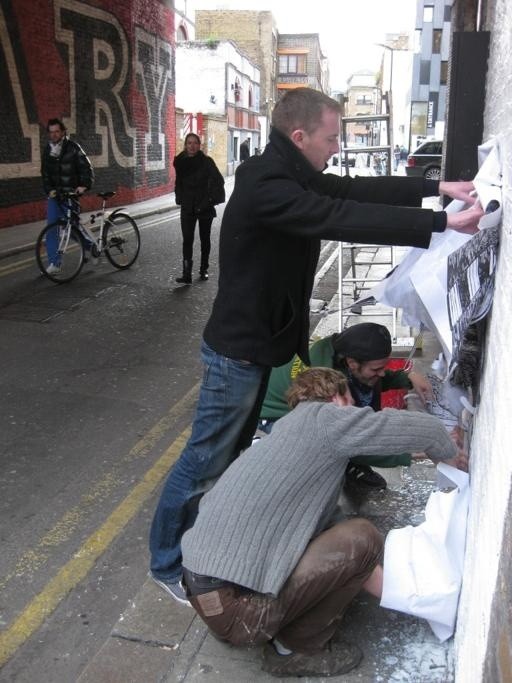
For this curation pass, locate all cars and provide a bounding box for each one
[333,146,355,167]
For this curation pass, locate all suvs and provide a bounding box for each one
[406,140,444,180]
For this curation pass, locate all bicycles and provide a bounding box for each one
[35,189,141,282]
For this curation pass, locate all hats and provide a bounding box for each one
[334,323,391,361]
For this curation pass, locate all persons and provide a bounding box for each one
[143,86,484,611]
[240,139,250,162]
[36,118,97,275]
[392,144,401,172]
[168,133,225,283]
[257,320,433,500]
[400,145,408,164]
[173,364,473,679]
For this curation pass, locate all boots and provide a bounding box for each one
[176,259,209,283]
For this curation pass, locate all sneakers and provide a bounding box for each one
[264,642,361,677]
[40,263,61,274]
[153,577,189,604]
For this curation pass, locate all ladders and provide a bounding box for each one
[335,90,398,344]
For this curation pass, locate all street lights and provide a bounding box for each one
[379,41,409,93]
